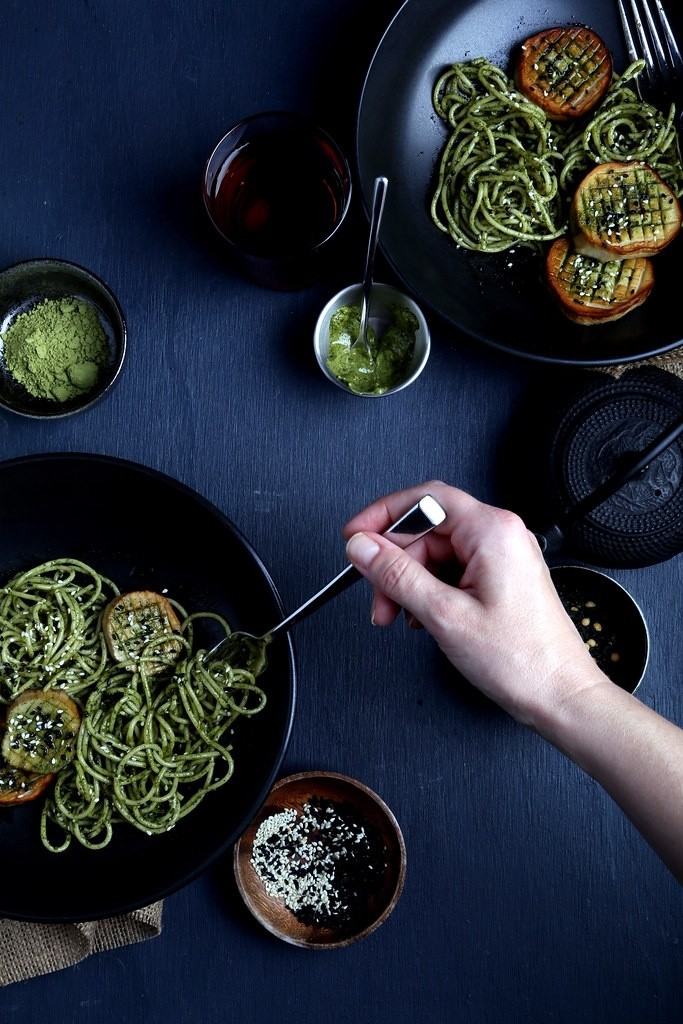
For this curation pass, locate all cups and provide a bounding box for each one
[0,260,128,422]
[313,283,432,398]
[201,113,352,272]
[545,565,649,696]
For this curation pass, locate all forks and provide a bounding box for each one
[198,493,450,690]
[618,0,682,147]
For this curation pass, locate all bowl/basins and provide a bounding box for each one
[233,770,406,950]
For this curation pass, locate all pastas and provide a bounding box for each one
[0,555,266,851]
[432,48,683,255]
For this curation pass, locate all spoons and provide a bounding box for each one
[351,176,386,359]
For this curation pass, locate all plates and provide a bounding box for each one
[352,0,682,368]
[0,448,298,926]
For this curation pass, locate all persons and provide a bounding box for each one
[342,479,683,884]
